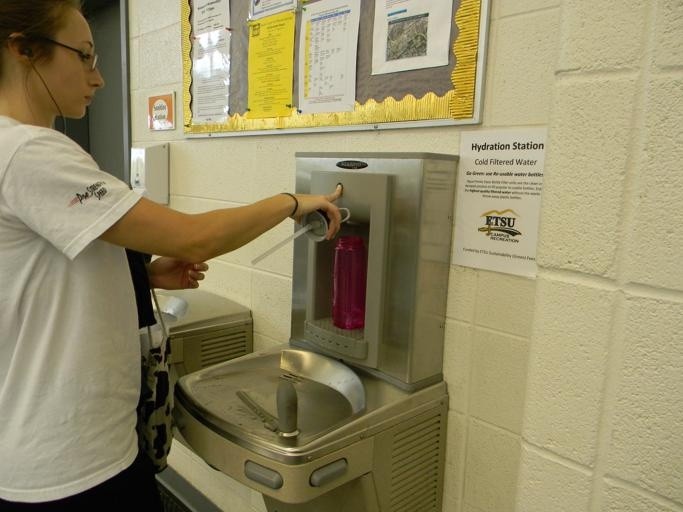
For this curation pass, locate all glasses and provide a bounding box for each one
[30,34,99,70]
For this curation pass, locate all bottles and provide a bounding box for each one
[331,236,368,330]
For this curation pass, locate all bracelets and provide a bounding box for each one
[281,192,299,216]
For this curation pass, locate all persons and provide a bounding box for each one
[2,0,344,512]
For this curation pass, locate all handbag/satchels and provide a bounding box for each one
[137,337,177,476]
